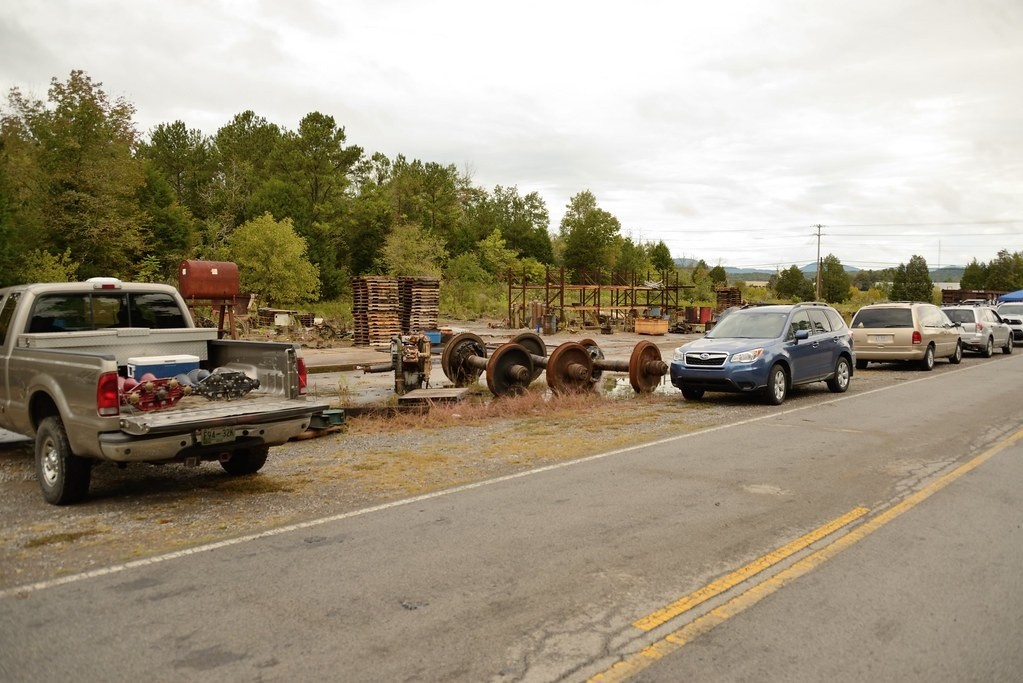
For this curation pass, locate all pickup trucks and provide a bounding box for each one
[0,276,332,505]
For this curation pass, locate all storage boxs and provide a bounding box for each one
[127,354,201,382]
[634,318,669,335]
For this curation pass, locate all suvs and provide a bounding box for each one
[847,301,963,370]
[667,300,856,408]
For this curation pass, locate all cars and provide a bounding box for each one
[941,301,1014,356]
[995,301,1023,342]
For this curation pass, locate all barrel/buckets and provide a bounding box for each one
[543,314,557,334]
[685,306,698,324]
[699,306,712,324]
